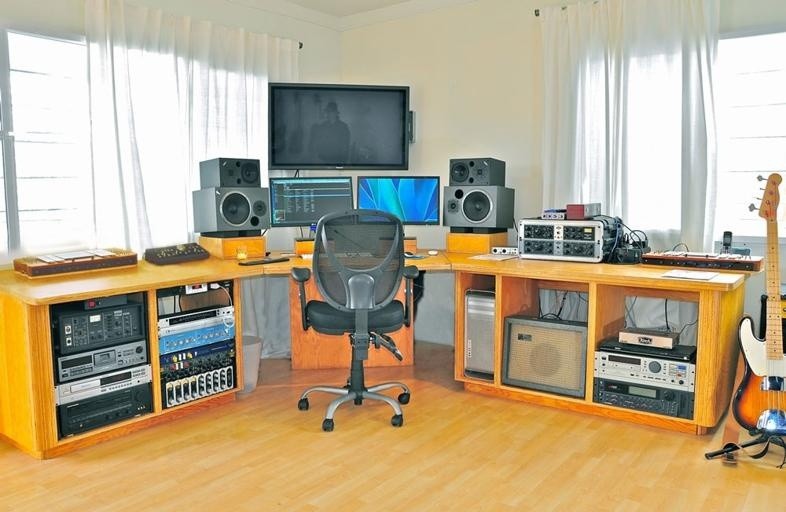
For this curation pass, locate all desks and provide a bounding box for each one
[263,250,452,370]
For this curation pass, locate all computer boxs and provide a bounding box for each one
[463,288,495,381]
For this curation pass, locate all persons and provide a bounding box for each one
[307,99,350,164]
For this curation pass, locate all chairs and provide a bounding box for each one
[290,209,419,431]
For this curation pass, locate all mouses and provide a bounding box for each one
[429,250,438,255]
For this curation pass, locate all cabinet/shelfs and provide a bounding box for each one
[444,250,751,436]
[1,258,264,460]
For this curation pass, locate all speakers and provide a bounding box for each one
[501,315,587,398]
[450,158,505,186]
[192,188,269,232]
[443,185,514,228]
[199,158,260,189]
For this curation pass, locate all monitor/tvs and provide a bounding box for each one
[269,177,354,241]
[356,177,439,240]
[268,83,408,169]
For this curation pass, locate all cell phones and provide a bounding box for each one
[404,252,413,258]
[724,232,732,246]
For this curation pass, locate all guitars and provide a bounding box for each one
[732,173,785,437]
